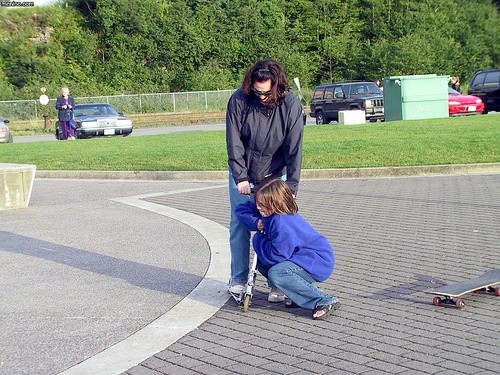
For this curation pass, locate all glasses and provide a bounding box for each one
[252,87,271,96]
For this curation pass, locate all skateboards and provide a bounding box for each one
[422,269,500,310]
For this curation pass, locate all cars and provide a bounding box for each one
[301,104,307,125]
[0,118,13,144]
[448,86,485,117]
[54,102,133,140]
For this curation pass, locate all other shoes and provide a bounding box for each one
[285,301,301,308]
[225,284,245,301]
[312,302,341,320]
[268,288,289,302]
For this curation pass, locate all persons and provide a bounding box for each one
[452,77,460,92]
[234,176,341,320]
[375,79,380,86]
[56,88,74,140]
[226,60,304,301]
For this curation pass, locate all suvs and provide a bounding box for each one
[468,68,500,115]
[309,80,385,125]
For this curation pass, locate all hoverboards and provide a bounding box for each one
[235,187,299,313]
